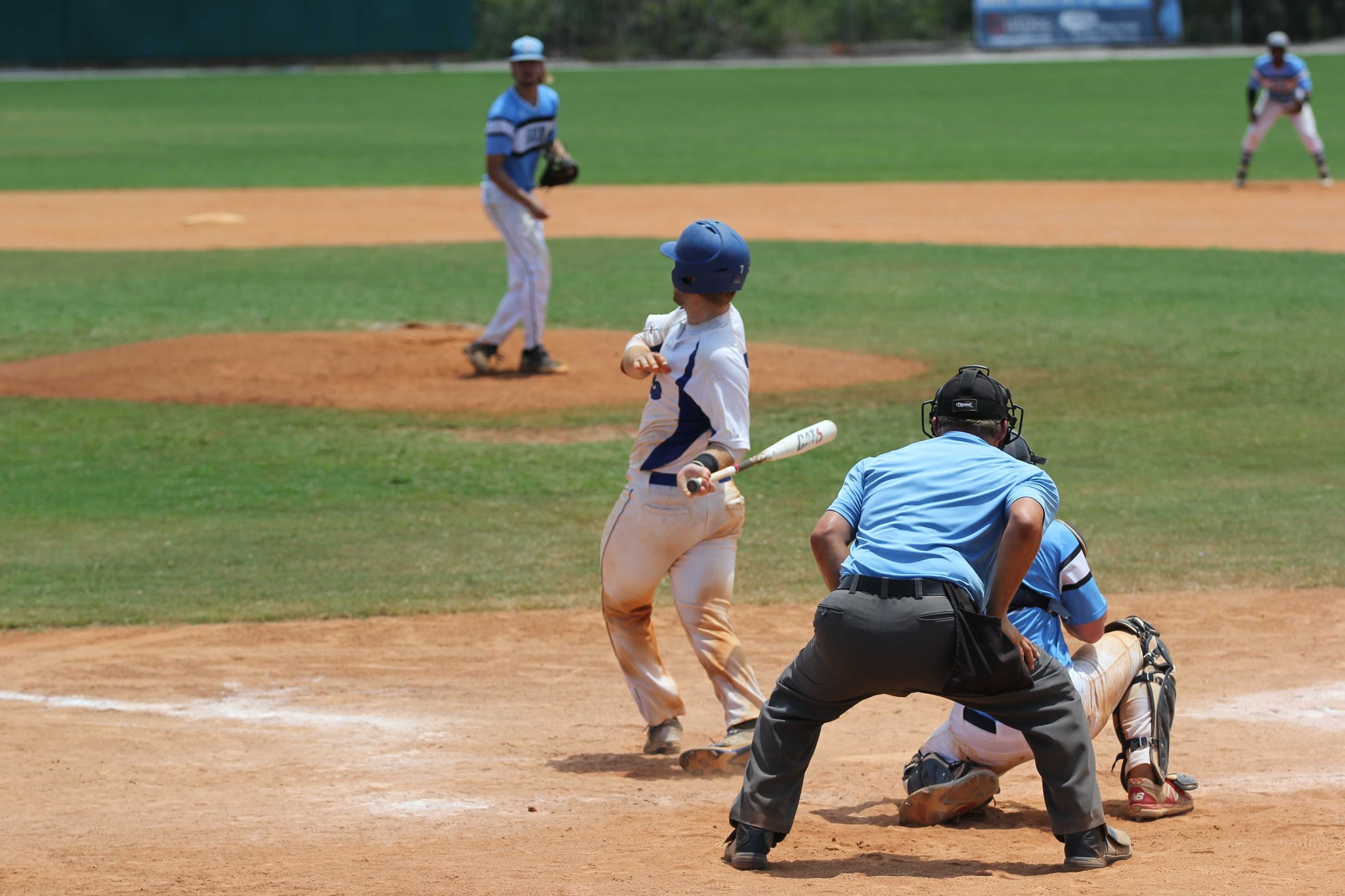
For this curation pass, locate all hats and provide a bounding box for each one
[929,365,1010,417]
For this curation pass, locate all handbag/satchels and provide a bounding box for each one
[942,607,1033,696]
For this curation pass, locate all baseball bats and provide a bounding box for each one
[686,419,838,493]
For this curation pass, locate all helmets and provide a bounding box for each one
[660,220,750,293]
[998,429,1047,466]
[507,35,544,62]
[1266,31,1292,50]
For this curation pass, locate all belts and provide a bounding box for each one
[649,473,731,487]
[963,707,996,734]
[834,575,945,597]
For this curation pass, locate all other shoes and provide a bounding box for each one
[1235,175,1246,186]
[1320,176,1335,188]
[1063,825,1133,872]
[722,823,775,870]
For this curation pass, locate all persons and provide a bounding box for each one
[598,222,766,781]
[899,427,1194,822]
[1236,30,1332,189]
[460,36,580,374]
[715,365,1131,870]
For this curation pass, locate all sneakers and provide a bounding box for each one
[678,722,766,780]
[899,766,999,827]
[462,340,504,375]
[517,344,569,374]
[1127,777,1194,820]
[643,719,683,754]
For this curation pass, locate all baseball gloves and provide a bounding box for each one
[1282,95,1303,114]
[539,154,580,187]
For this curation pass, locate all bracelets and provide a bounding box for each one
[689,453,719,474]
